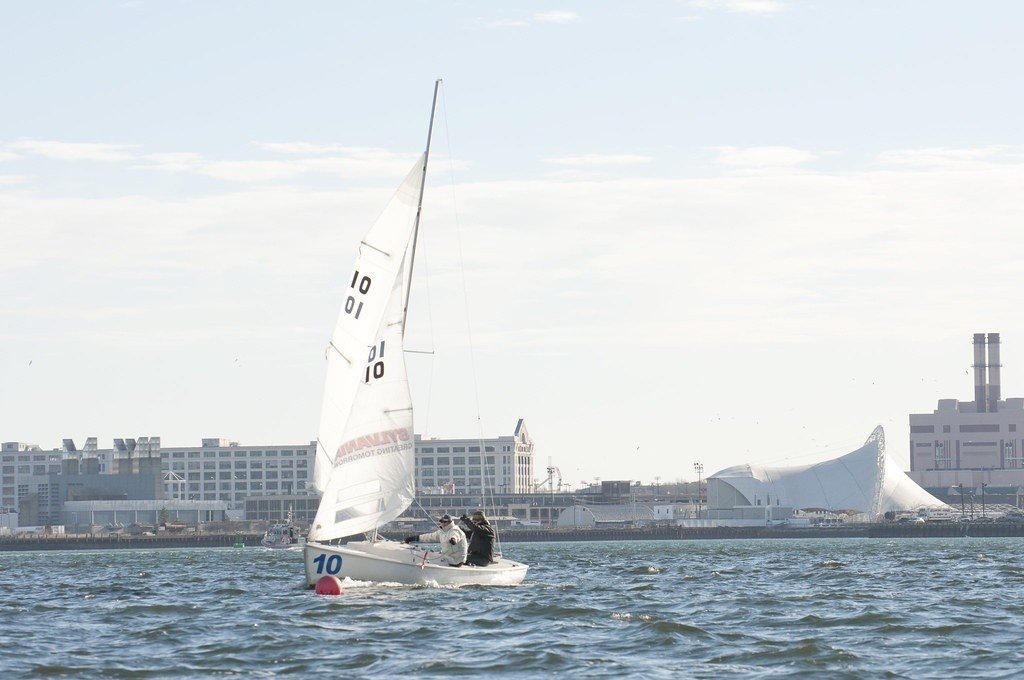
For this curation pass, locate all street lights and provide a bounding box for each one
[694,462,703,517]
[982,482,987,517]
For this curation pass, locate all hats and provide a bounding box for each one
[439,514,452,522]
[471,511,485,522]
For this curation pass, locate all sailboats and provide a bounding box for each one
[305,76,531,597]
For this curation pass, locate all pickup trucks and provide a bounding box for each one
[974,517,993,523]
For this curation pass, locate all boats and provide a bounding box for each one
[261,509,306,551]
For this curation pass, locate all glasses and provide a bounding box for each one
[441,521,447,523]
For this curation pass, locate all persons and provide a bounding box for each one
[405,513,467,568]
[459,511,496,567]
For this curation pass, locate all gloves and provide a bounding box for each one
[405,536,419,543]
[461,515,468,521]
[450,538,455,545]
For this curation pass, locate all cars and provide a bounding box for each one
[909,518,924,523]
[958,515,971,522]
[996,517,1011,524]
